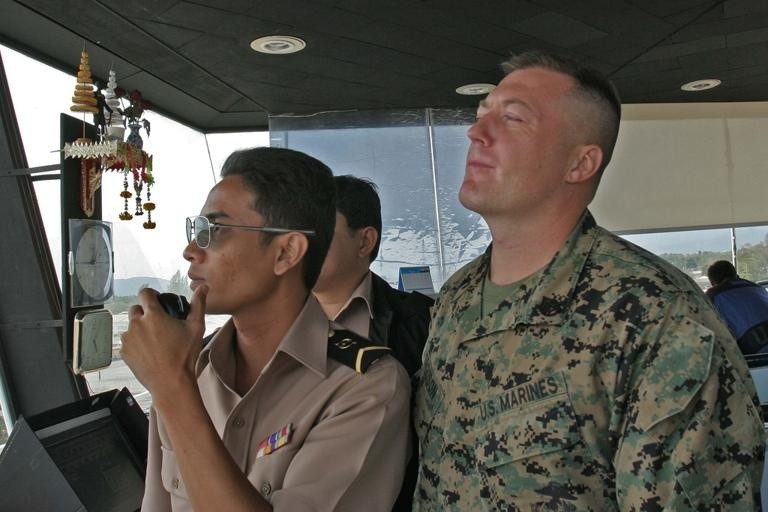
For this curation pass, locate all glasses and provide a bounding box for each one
[186,216,317,250]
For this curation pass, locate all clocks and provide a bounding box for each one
[69,218,116,376]
